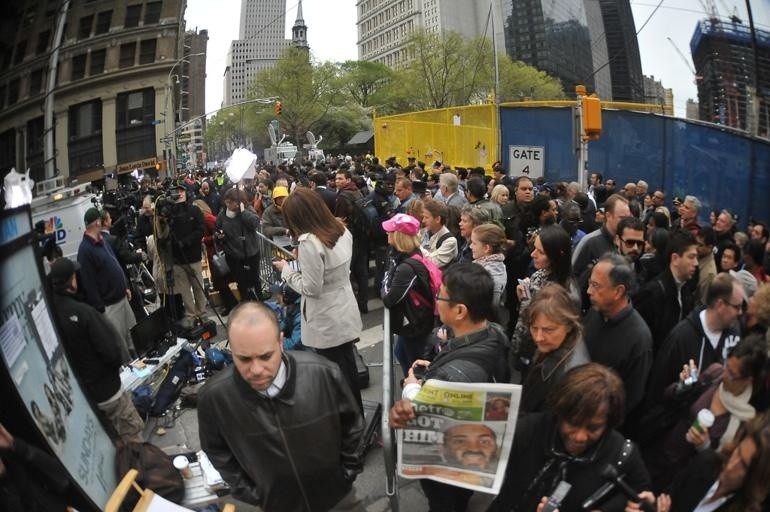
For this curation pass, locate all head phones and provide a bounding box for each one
[151,195,155,208]
[181,183,191,200]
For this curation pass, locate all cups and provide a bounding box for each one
[692,408,715,435]
[173,455,193,479]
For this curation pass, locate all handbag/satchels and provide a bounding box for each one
[210,251,232,279]
[112,439,185,507]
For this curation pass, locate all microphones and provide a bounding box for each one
[602,464,655,512]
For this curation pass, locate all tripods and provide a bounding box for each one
[122,241,155,316]
[168,220,228,329]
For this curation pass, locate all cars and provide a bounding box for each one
[206,159,229,172]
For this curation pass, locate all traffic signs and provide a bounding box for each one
[160,137,174,143]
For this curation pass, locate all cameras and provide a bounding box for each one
[400,365,426,390]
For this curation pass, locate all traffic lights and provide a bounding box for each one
[276,104,282,115]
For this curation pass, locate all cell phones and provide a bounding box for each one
[541,481,572,512]
[216,227,221,235]
[272,256,281,262]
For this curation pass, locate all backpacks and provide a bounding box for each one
[341,188,380,237]
[435,233,465,275]
[408,252,444,317]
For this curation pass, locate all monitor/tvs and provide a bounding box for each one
[129,307,171,359]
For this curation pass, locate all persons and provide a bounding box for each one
[0,150,769,512]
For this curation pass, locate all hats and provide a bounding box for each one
[672,196,684,205]
[387,156,442,172]
[83,207,101,223]
[49,257,82,285]
[176,182,190,191]
[380,211,419,237]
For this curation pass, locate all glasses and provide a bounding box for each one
[586,277,617,292]
[620,236,645,250]
[723,298,744,312]
[551,419,606,466]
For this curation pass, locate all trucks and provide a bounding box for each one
[262,119,326,168]
[32,175,105,275]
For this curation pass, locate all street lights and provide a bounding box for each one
[229,112,242,146]
[160,51,206,177]
[219,121,226,158]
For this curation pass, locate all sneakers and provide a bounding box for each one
[220,302,239,317]
[355,297,370,313]
